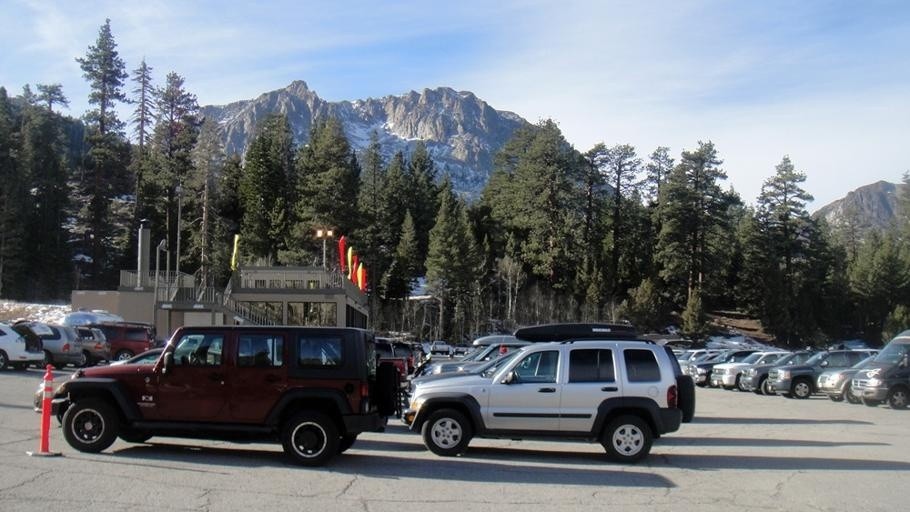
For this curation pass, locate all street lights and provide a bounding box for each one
[316,228,334,270]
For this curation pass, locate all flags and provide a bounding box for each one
[338,235,367,290]
[230,233,239,272]
